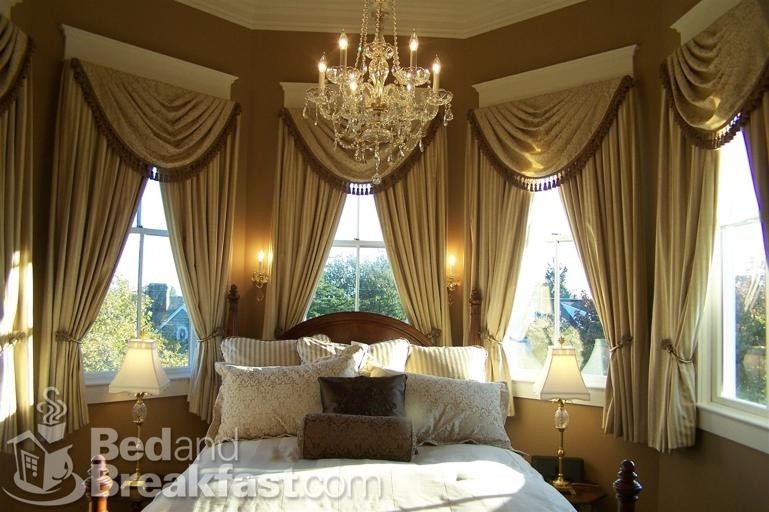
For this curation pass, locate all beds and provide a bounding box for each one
[81,284,642,512]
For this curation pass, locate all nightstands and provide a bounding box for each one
[106,475,164,512]
[530,455,606,512]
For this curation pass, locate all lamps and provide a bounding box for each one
[444,254,460,307]
[107,328,171,489]
[251,250,268,304]
[302,0,454,186]
[533,334,591,497]
